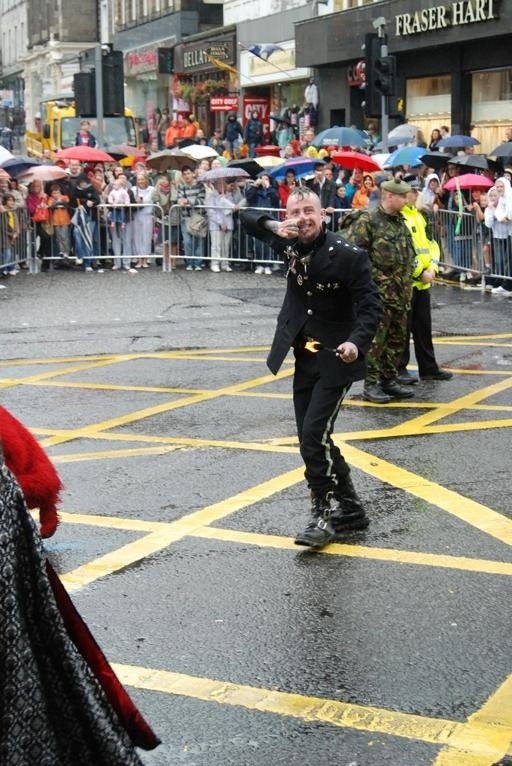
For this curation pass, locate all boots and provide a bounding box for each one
[329,475,371,533]
[294,486,338,549]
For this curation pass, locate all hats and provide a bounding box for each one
[380,172,421,195]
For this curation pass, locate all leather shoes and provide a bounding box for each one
[377,380,414,398]
[362,385,391,404]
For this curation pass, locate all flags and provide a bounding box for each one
[248,43,285,61]
[205,54,237,86]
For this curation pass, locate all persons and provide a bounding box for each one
[236,186,382,547]
[347,176,415,401]
[394,172,454,386]
[0,405,162,764]
[1,77,511,304]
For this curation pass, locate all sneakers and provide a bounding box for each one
[396,375,418,385]
[253,265,283,276]
[439,266,512,296]
[415,370,453,382]
[84,263,233,276]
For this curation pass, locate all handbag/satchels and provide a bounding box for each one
[32,206,50,223]
[185,213,209,239]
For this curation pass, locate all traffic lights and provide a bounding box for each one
[373,56,391,91]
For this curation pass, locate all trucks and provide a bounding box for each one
[22,97,138,167]
